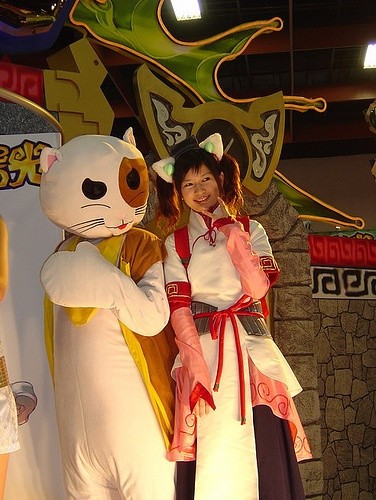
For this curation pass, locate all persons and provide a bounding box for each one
[0,340,20,500]
[152,133,314,500]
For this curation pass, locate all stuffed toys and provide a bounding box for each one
[39,128,187,500]
